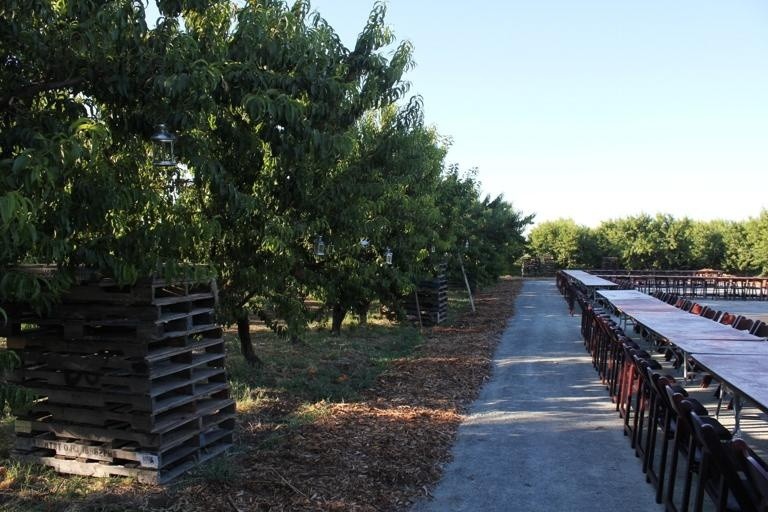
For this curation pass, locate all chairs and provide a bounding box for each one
[554,269,768,512]
